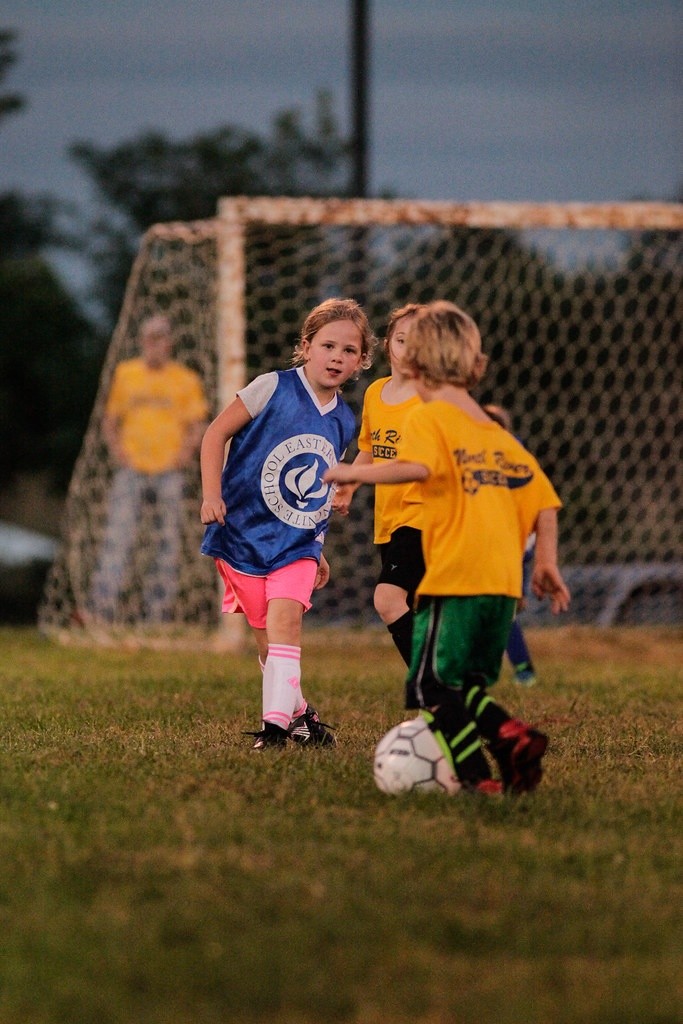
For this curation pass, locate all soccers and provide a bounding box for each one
[371,716,461,798]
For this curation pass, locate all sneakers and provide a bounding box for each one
[242,720,287,752]
[484,718,549,800]
[514,663,535,689]
[287,704,336,750]
[468,780,501,803]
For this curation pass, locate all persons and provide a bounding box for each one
[331,304,424,668]
[483,405,535,686]
[88,314,211,631]
[199,297,374,751]
[322,302,569,798]
